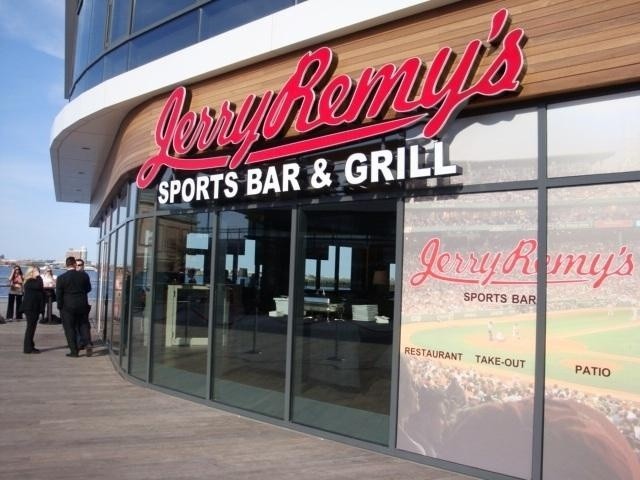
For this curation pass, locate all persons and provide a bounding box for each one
[188,267,197,285]
[39,268,58,325]
[225,270,230,282]
[7,265,25,321]
[22,267,45,355]
[57,256,93,358]
[75,258,91,350]
[0,314,6,326]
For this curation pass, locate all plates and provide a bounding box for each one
[352,304,379,322]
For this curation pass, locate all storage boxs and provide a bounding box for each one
[273,296,289,316]
[351,303,379,323]
